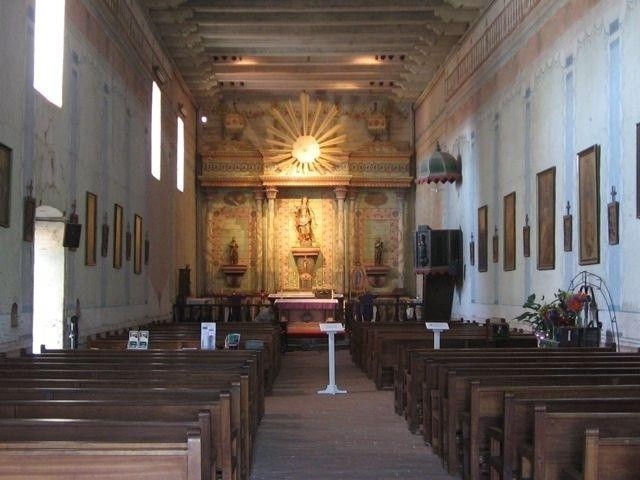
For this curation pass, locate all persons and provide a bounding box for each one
[374,237,385,265]
[227,236,239,265]
[293,196,318,245]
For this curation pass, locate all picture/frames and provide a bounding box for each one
[84,191,142,275]
[0,144,15,226]
[476,144,599,274]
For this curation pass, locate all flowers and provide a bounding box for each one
[513,287,593,337]
